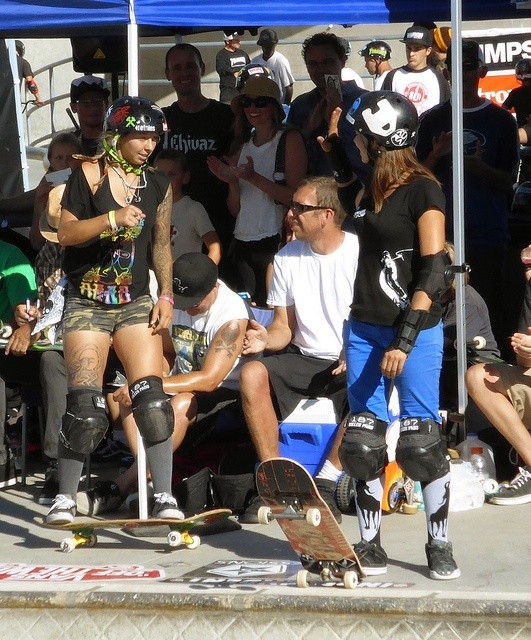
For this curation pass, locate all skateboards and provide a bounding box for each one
[256,457,365,590]
[33,509,233,555]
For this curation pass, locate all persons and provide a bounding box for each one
[116,251,250,513]
[27,132,85,278]
[345,90,460,582]
[63,76,111,162]
[150,152,220,271]
[43,95,186,524]
[206,75,327,92]
[1,237,70,507]
[418,40,522,347]
[285,32,373,178]
[239,175,359,524]
[380,26,444,117]
[464,243,531,506]
[159,43,239,293]
[356,40,392,91]
[12,40,45,107]
[215,34,249,104]
[205,75,309,313]
[249,29,293,106]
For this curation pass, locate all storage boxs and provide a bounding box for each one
[278,387,399,482]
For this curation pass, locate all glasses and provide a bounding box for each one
[239,96,273,107]
[289,200,335,212]
[448,61,479,71]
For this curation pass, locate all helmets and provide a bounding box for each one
[257,29,278,44]
[103,96,168,132]
[235,64,274,91]
[359,41,391,59]
[346,91,419,148]
[15,40,25,57]
[515,59,531,85]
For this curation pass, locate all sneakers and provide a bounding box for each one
[0,460,16,488]
[353,540,388,576]
[152,492,184,520]
[426,539,460,579]
[126,481,155,514]
[39,460,58,505]
[314,479,342,523]
[76,481,121,515]
[46,494,75,524]
[488,466,531,505]
[244,493,266,523]
[94,438,131,461]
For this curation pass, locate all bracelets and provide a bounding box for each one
[158,296,173,306]
[107,210,118,232]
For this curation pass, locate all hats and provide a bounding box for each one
[223,32,238,40]
[71,76,110,102]
[399,26,432,46]
[40,184,66,242]
[231,77,286,121]
[445,40,483,64]
[434,27,451,52]
[157,252,218,309]
